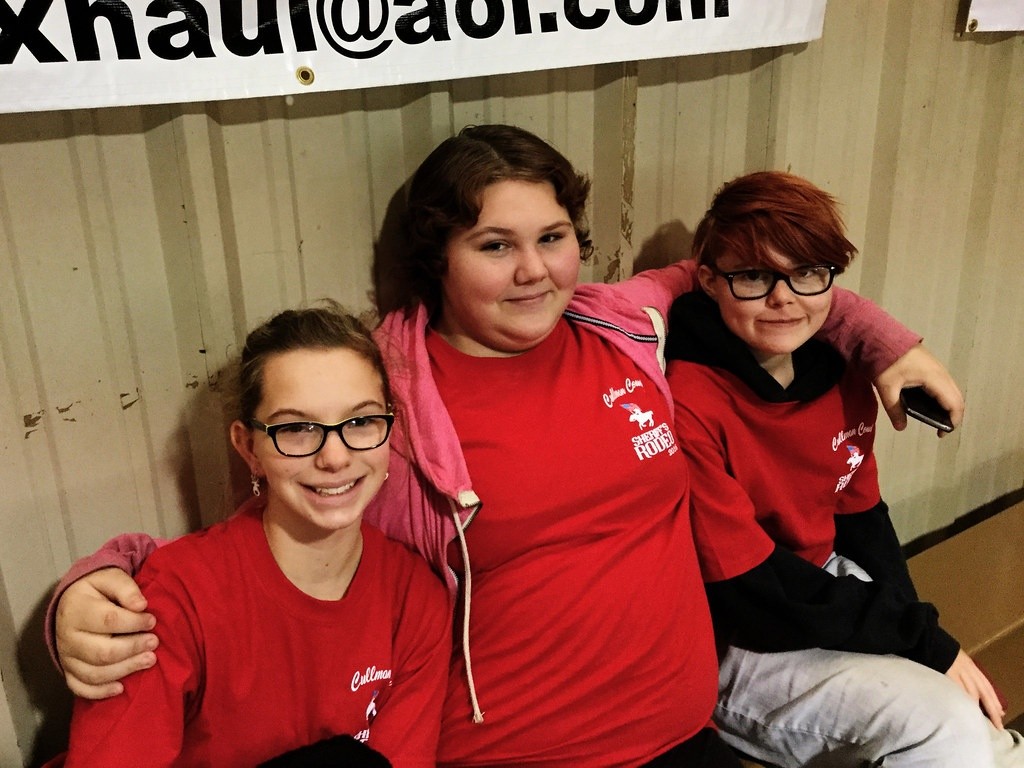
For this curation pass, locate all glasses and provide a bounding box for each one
[701,258,841,300]
[246,402,394,457]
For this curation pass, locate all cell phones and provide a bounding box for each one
[899,385,955,433]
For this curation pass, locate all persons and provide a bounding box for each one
[667,171,1024,768]
[46,122,962,768]
[40,298,454,768]
